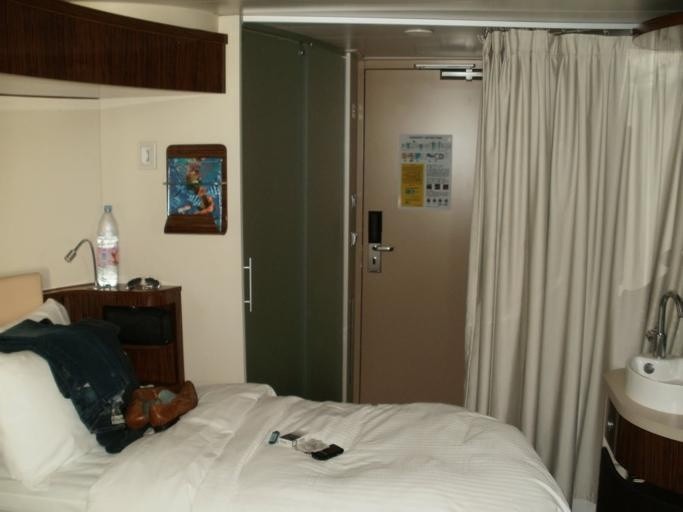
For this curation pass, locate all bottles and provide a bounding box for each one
[95,205,121,287]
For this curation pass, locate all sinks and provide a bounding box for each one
[625,352,683,415]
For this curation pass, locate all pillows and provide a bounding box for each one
[0,298,97,490]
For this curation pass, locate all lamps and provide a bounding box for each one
[413,64,475,68]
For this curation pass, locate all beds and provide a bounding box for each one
[0,268,572,512]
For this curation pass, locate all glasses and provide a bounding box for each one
[125,274,161,292]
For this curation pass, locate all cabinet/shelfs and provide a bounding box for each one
[597,399,683,511]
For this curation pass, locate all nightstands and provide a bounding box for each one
[44,282,185,384]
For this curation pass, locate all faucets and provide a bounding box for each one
[655,290,682,359]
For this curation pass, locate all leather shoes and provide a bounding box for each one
[126,376,197,434]
